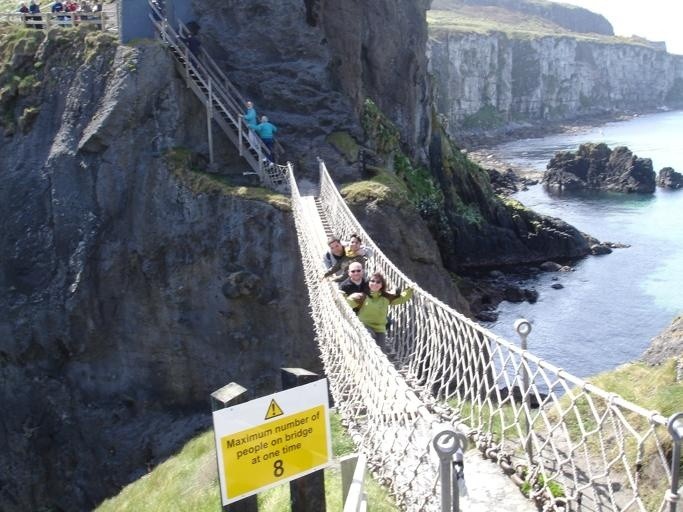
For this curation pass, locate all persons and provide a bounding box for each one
[20,1,82,30]
[238,101,256,150]
[179,34,200,67]
[248,115,277,168]
[151,0,167,21]
[318,234,415,350]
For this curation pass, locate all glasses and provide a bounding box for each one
[369,280,382,284]
[350,270,362,273]
